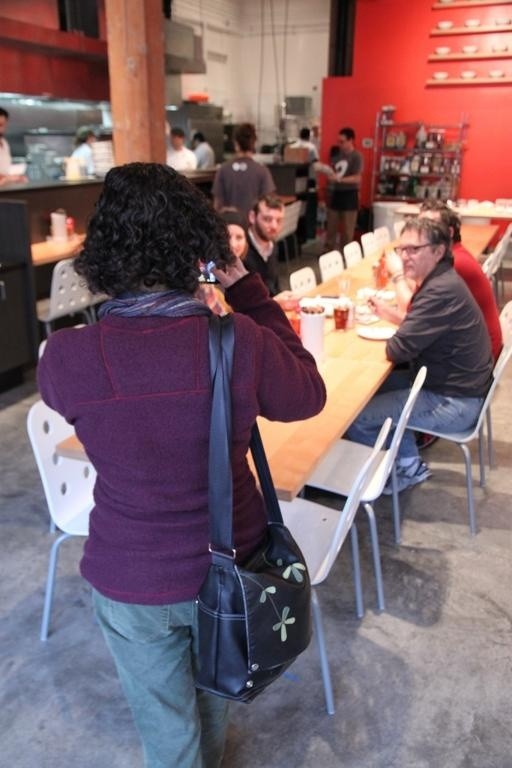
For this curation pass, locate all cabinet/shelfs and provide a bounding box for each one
[372,106,468,204]
[425,0,512,86]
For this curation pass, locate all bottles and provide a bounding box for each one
[376,256,389,288]
[279,292,326,368]
[51,207,75,243]
[378,124,456,198]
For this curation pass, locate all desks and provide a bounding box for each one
[57,225,501,500]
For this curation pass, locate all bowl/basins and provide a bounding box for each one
[432,16,511,81]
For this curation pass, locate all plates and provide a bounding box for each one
[358,327,397,339]
[90,139,113,177]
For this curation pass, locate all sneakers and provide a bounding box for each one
[380,455,434,496]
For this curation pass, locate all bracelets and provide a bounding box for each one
[393,272,404,282]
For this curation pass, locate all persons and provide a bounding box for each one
[380,198,501,449]
[92,133,114,177]
[165,127,198,172]
[348,216,495,496]
[37,161,327,768]
[213,125,276,215]
[66,131,97,176]
[318,127,362,258]
[292,128,319,162]
[216,211,246,258]
[192,132,215,169]
[0,108,29,187]
[215,125,236,163]
[242,193,284,296]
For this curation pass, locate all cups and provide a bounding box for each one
[332,304,357,330]
[495,197,512,212]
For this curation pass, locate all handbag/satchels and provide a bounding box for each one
[192,516,317,705]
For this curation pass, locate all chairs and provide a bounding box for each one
[298,364,428,607]
[26,199,512,334]
[381,342,510,541]
[25,400,98,643]
[278,417,393,714]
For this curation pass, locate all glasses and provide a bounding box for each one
[392,242,437,257]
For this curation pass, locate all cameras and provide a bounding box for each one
[196,254,226,283]
